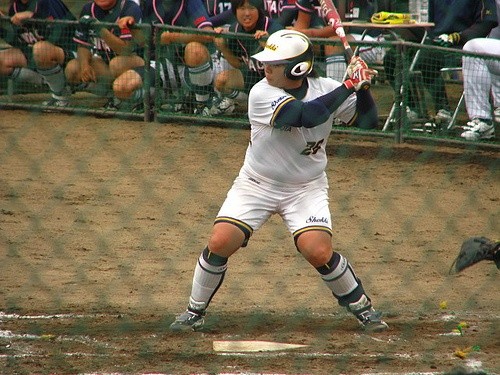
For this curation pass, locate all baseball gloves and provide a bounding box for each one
[455,236,500,273]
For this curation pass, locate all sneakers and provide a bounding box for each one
[411,117,429,132]
[425,110,456,132]
[448,238,493,275]
[93,98,130,118]
[210,96,235,115]
[190,105,212,122]
[125,103,157,121]
[170,305,205,332]
[447,117,495,141]
[39,94,74,113]
[348,294,392,334]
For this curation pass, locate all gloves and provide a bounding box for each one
[80,15,100,30]
[343,68,379,92]
[432,32,455,53]
[345,56,369,77]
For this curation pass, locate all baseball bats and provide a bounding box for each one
[318,0,370,91]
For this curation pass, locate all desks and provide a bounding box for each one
[339,21,436,132]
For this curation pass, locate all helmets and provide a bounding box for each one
[250,29,315,80]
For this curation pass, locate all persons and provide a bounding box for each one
[0,0,500,142]
[170,29,388,333]
[455,237,500,273]
